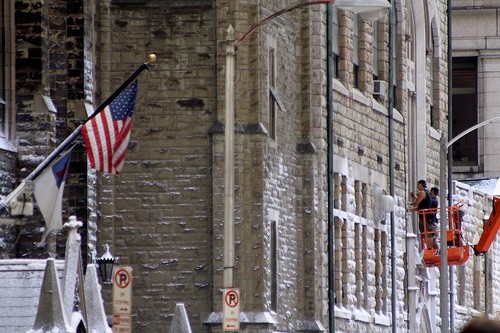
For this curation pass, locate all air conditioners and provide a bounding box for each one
[373,80,389,96]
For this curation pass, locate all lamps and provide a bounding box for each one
[95,244,116,285]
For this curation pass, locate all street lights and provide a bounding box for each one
[222,0,392,333]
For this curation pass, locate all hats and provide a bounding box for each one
[418,180,427,188]
